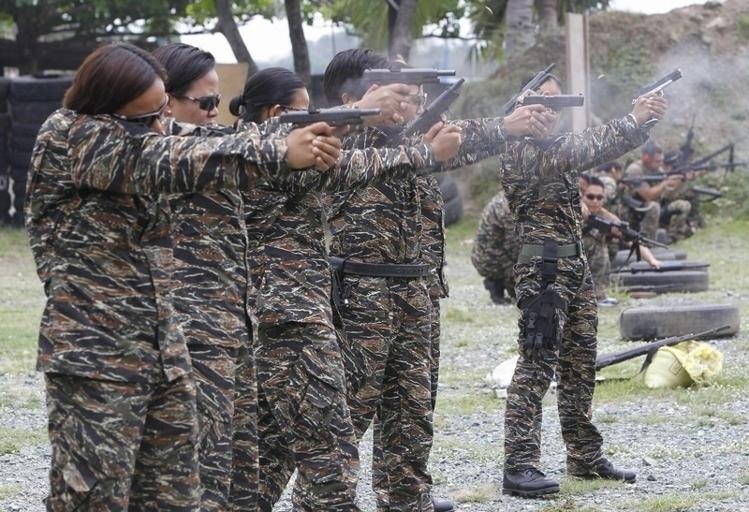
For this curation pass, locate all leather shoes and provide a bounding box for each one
[484,278,512,306]
[502,468,561,498]
[583,459,637,484]
[434,500,454,511]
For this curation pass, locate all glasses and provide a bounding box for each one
[172,93,223,112]
[584,193,604,201]
[280,106,321,115]
[112,93,170,129]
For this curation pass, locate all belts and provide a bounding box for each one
[518,244,578,260]
[329,256,430,278]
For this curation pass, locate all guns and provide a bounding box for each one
[503,62,556,114]
[359,65,455,88]
[523,92,584,113]
[629,68,682,128]
[404,78,466,136]
[604,264,710,276]
[620,113,747,190]
[552,323,730,381]
[279,104,382,136]
[582,216,670,265]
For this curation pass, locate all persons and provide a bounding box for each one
[228,67,466,510]
[27,42,350,511]
[322,47,562,512]
[153,42,430,511]
[499,71,668,498]
[470,142,706,305]
